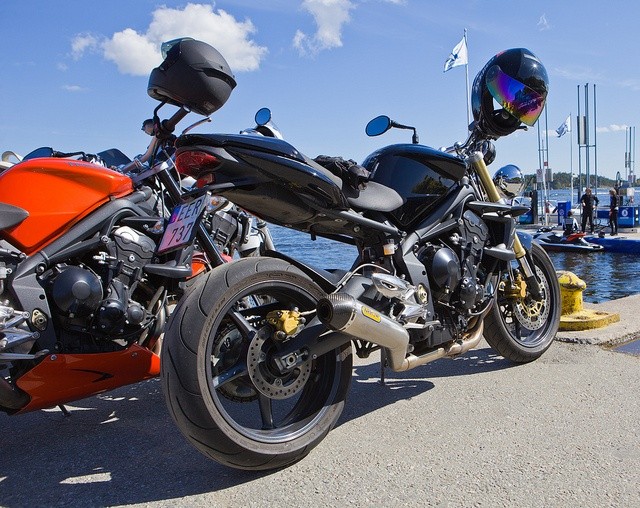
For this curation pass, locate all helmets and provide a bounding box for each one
[472,47,550,138]
[147,37,237,116]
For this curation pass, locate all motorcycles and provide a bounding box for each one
[2,94,270,418]
[154,97,562,472]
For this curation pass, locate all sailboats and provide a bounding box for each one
[515,82,640,226]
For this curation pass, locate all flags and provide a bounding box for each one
[443,38,467,72]
[555,115,571,138]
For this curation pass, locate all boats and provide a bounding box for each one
[533,225,604,254]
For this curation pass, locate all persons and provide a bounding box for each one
[580,188,599,235]
[562,211,580,238]
[608,189,620,236]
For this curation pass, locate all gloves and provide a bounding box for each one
[313,155,372,191]
